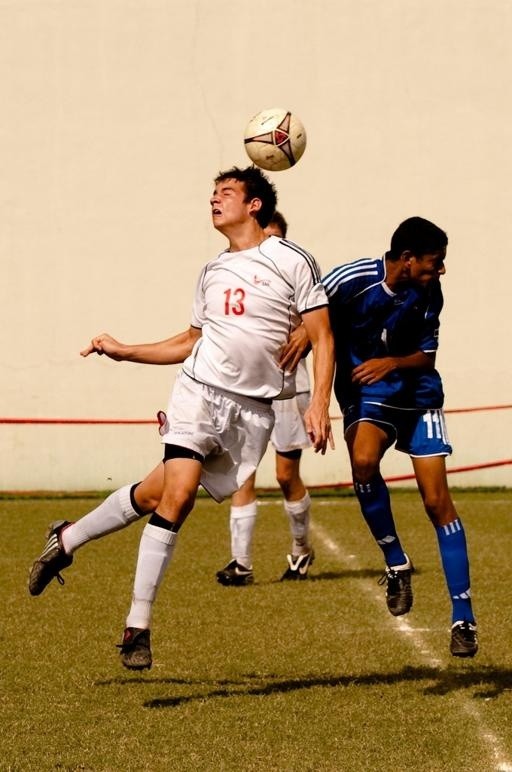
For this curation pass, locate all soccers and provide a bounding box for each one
[244,109,306,171]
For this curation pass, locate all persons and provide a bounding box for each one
[28,163,337,671]
[276,215,480,657]
[213,211,316,585]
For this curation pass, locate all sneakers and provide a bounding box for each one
[215,558,255,585]
[116,624,152,671]
[280,544,315,581]
[449,619,478,656]
[377,552,414,616]
[28,519,74,596]
